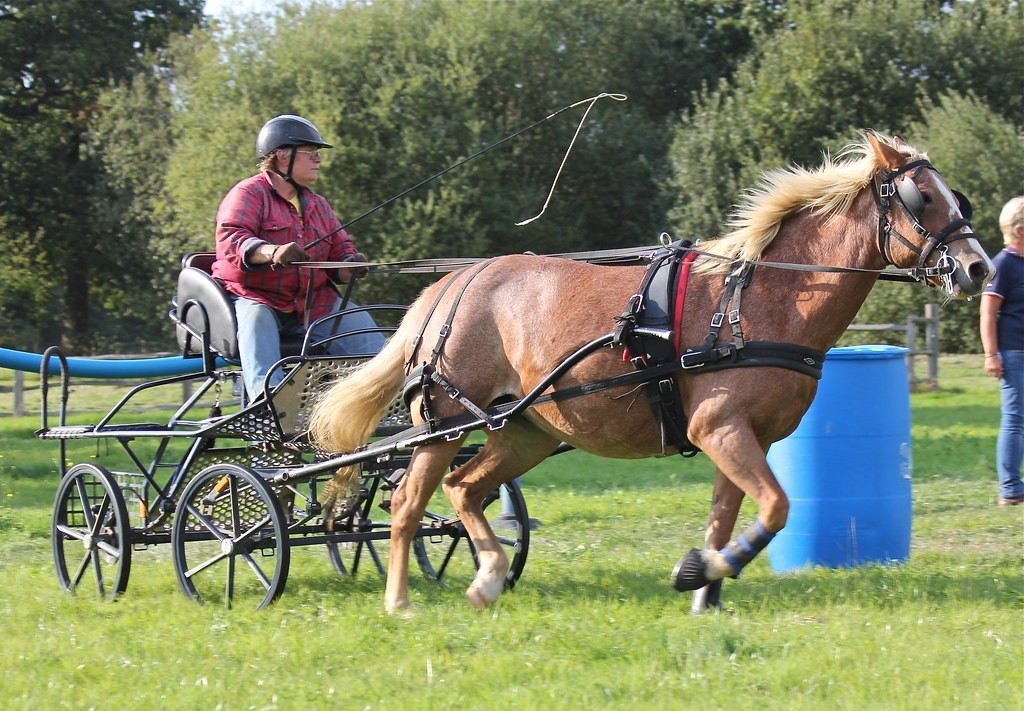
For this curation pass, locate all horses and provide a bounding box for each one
[308,128,995,621]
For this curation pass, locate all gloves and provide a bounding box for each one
[338,253,369,285]
[272,241,312,270]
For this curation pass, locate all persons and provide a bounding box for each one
[211,114,387,444]
[980,195,1024,506]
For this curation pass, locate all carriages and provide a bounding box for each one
[34,128,997,621]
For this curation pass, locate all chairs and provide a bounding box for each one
[176,251,322,359]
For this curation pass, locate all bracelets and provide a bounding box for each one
[269,246,280,260]
[984,353,998,357]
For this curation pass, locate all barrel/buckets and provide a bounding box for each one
[765,344,914,569]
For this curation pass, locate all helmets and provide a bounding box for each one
[255,114,334,159]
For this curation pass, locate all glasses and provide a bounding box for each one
[296,148,320,158]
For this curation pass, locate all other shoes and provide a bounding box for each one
[998,493,1024,507]
